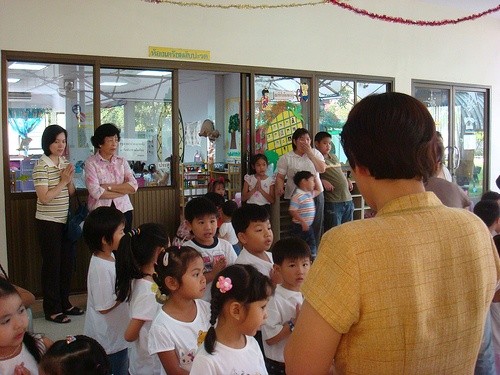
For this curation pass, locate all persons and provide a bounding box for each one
[284,92,500,375]
[424,138,474,213]
[433,130,452,184]
[314,130,354,241]
[130,156,181,188]
[84,123,139,232]
[32,124,85,324]
[275,127,324,259]
[239,154,276,211]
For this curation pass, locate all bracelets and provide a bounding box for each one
[0,161,500,375]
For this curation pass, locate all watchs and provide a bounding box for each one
[288,319,295,331]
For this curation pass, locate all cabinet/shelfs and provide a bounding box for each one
[179,163,241,220]
[270,180,374,250]
[10,158,39,191]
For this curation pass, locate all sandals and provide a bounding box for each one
[45,313,71,324]
[62,305,84,316]
